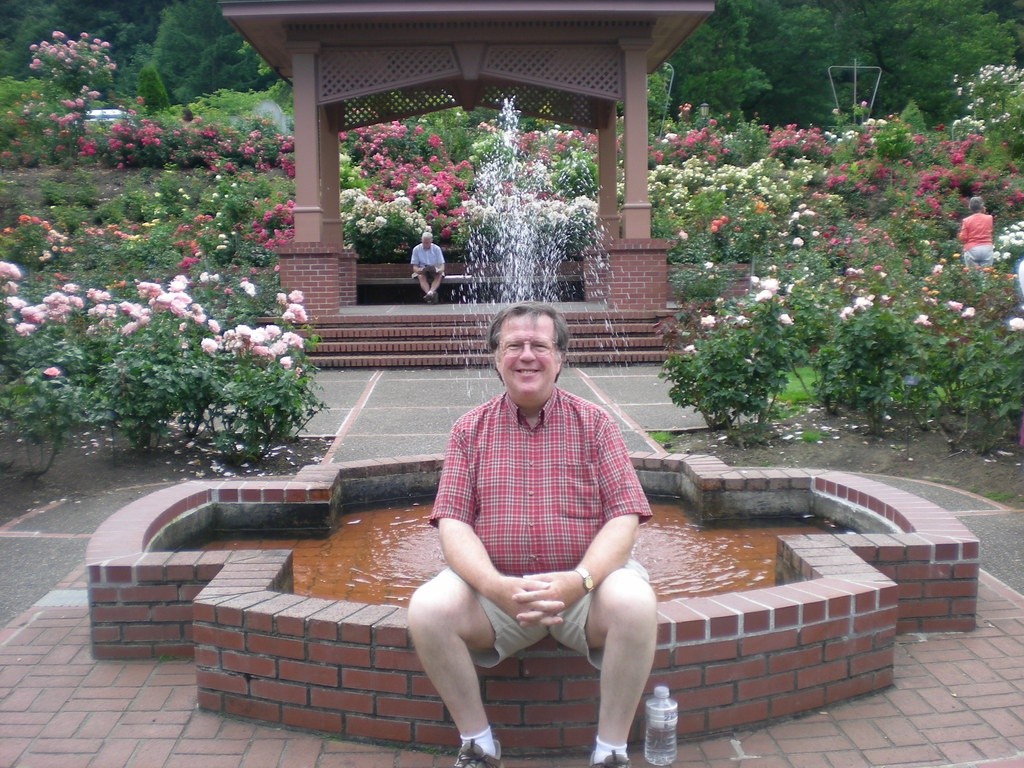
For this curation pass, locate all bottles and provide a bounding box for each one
[645,685,678,765]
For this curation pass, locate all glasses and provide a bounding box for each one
[500,336,558,358]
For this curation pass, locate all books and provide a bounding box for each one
[421,265,443,274]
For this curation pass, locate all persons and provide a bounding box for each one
[410,231,445,304]
[407,302,659,767]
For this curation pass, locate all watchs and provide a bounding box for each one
[573,565,595,595]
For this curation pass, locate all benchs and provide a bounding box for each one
[355,261,584,286]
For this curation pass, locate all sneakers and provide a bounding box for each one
[452,739,504,768]
[432,292,438,304]
[589,751,632,767]
[424,291,433,300]
